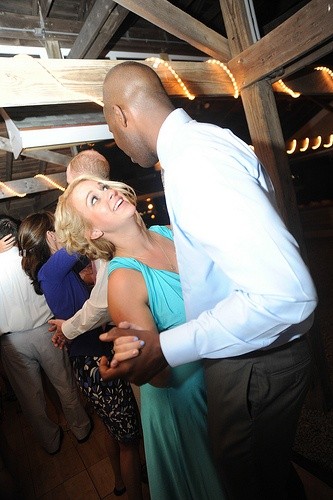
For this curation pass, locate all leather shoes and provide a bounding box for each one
[45,415,93,456]
[114,486,126,496]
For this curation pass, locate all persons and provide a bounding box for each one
[54,176,226,500]
[98,61,319,500]
[0,233,16,253]
[0,218,93,455]
[17,211,140,500]
[46,150,147,351]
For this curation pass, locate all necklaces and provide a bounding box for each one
[117,237,176,271]
